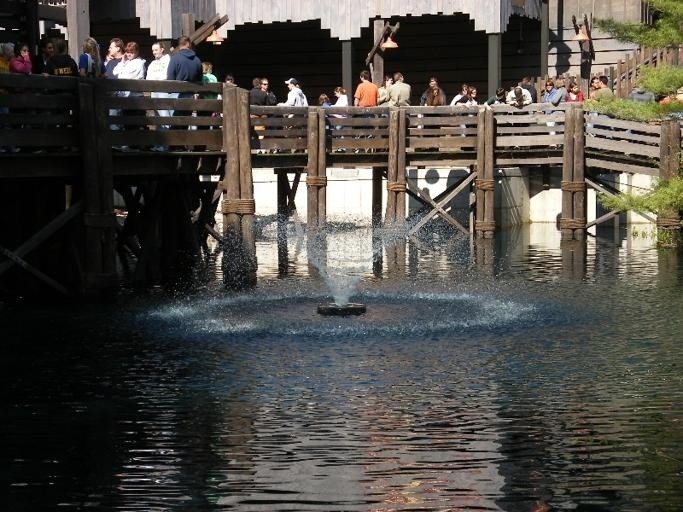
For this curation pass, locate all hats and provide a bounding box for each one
[284,77,297,86]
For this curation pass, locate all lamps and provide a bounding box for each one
[380,33,398,51]
[572,28,589,41]
[206,21,224,41]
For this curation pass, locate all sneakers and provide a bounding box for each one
[149,146,171,152]
[253,148,374,154]
[31,148,48,156]
[120,145,139,153]
[9,148,21,153]
[0,147,6,153]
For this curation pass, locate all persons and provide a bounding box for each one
[1,35,219,151]
[631,85,680,159]
[319,71,412,153]
[420,75,615,152]
[217,75,308,154]
